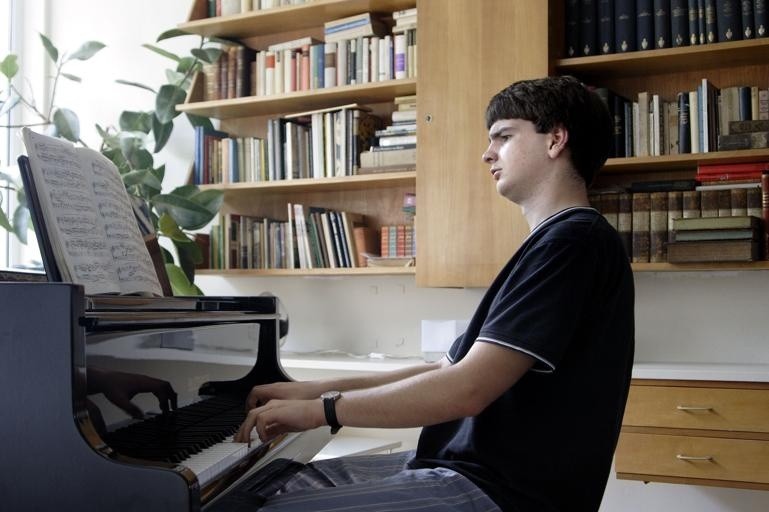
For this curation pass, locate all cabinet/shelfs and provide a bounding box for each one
[549,37,769,270]
[176,1,416,276]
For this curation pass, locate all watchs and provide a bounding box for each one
[320,391,342,435]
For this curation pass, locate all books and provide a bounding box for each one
[195,194,417,269]
[203,8,417,101]
[196,95,416,186]
[581,78,769,157]
[196,1,288,19]
[22,127,164,299]
[555,1,769,58]
[588,162,769,265]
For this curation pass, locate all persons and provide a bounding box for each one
[234,76,635,512]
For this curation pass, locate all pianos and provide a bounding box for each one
[2,282,338,511]
